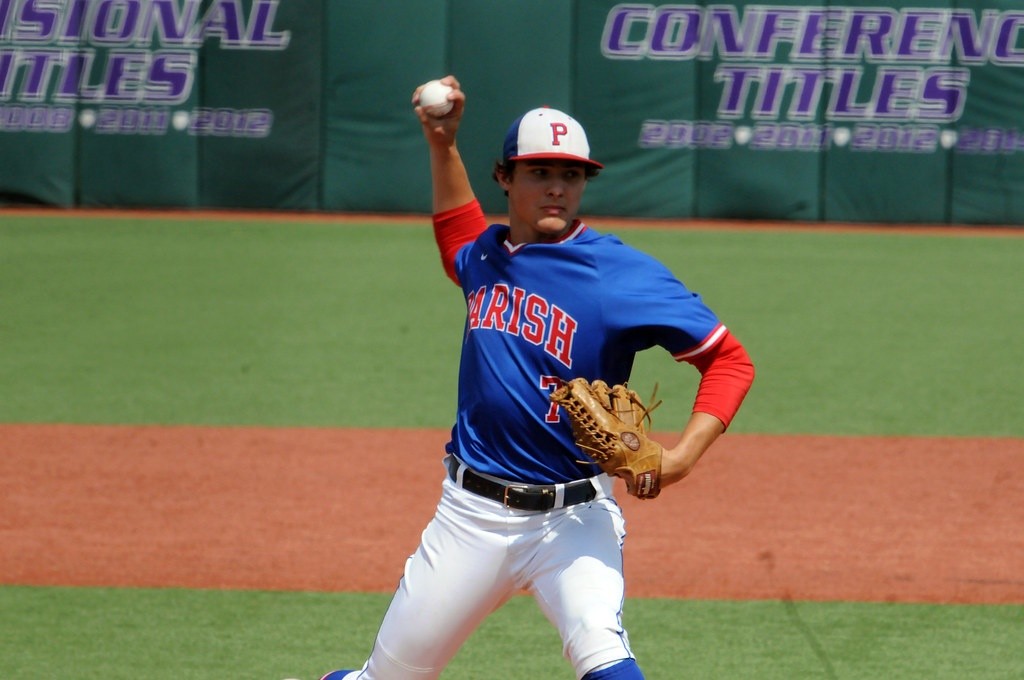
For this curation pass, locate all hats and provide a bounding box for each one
[503,106,604,170]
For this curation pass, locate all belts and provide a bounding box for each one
[448,454,595,511]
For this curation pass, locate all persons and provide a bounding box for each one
[283,75,756,680]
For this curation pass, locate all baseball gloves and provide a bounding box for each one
[548,377,663,501]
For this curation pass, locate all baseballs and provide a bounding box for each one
[419,80,455,116]
